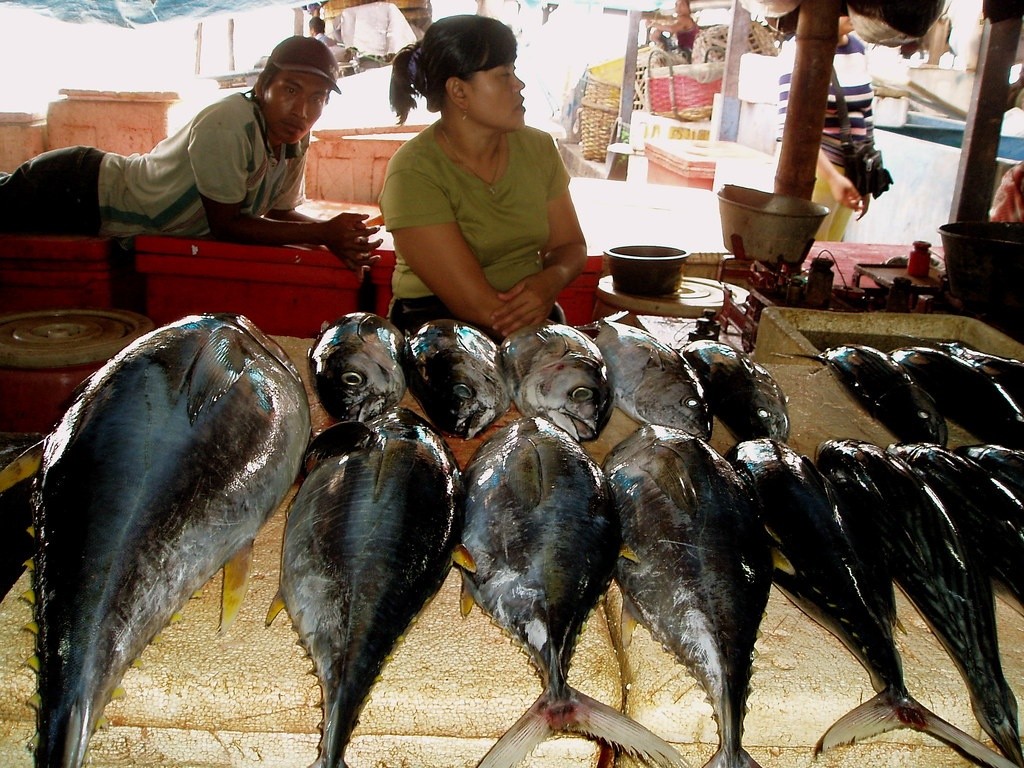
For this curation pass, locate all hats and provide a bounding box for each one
[270,35,342,95]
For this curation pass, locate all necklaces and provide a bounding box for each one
[438,117,499,195]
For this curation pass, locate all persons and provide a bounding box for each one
[376,13,587,347]
[644,0,699,64]
[308,17,343,63]
[0,36,384,272]
[778,13,874,242]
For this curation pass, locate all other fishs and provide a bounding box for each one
[2,311,1024,768]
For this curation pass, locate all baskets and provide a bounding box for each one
[591,58,625,85]
[583,74,621,108]
[644,47,725,120]
[579,105,618,162]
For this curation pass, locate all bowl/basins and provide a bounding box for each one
[716,182,832,266]
[937,221,1024,303]
[603,244,691,297]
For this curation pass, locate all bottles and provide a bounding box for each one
[785,256,834,309]
[908,241,932,277]
[886,276,912,314]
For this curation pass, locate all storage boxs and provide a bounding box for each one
[0,205,1024,768]
[646,136,764,190]
[0,84,179,174]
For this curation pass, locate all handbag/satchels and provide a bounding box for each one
[845,142,893,199]
